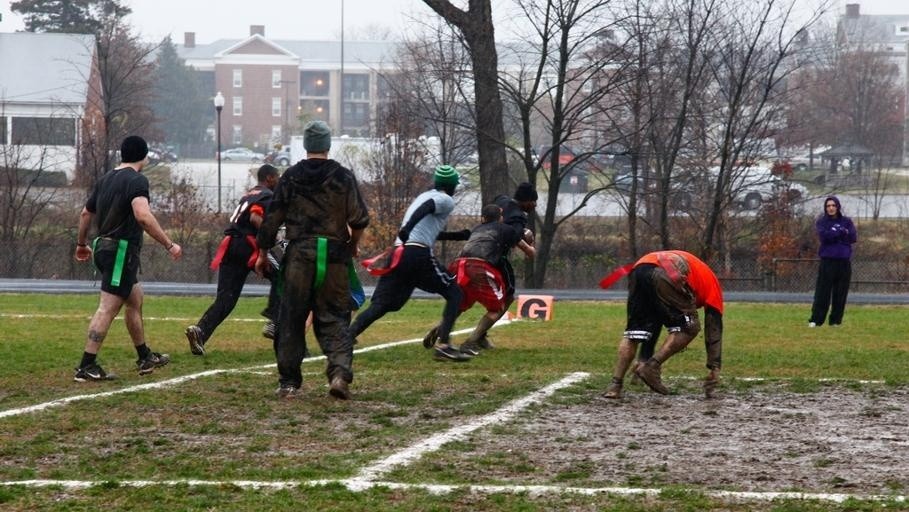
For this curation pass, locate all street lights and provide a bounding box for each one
[211,90,226,217]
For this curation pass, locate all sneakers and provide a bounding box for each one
[635,357,667,396]
[260,323,276,338]
[134,353,173,376]
[182,325,206,355]
[601,377,623,400]
[433,345,474,361]
[480,339,497,350]
[72,365,117,384]
[273,383,298,399]
[462,343,482,356]
[423,325,438,349]
[330,378,349,399]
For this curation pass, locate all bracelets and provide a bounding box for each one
[76,242,88,246]
[166,241,174,250]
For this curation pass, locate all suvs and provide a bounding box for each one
[274,144,292,167]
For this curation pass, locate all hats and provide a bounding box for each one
[432,161,463,188]
[481,205,500,218]
[513,181,539,200]
[118,135,149,163]
[302,120,333,153]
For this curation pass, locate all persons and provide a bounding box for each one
[254,120,369,401]
[349,164,473,363]
[808,196,856,328]
[184,164,288,356]
[423,203,537,356]
[484,182,538,322]
[302,227,366,358]
[601,250,724,399]
[74,135,182,384]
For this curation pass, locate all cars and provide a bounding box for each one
[613,165,806,210]
[506,140,576,171]
[215,148,265,163]
[148,148,178,163]
